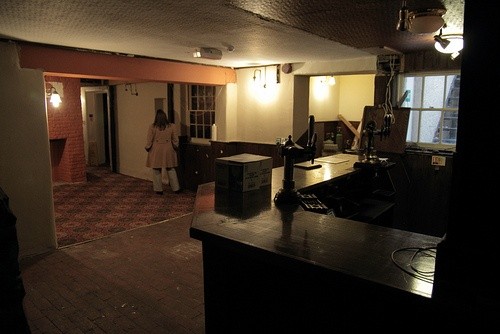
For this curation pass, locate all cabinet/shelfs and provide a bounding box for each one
[178,141,283,191]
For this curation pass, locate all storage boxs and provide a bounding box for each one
[215,157,261,192]
[232,153,272,186]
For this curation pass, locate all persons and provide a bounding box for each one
[0,185,31,334]
[146,109,180,193]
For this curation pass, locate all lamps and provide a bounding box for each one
[434,29,450,49]
[396,0,412,32]
[49,87,62,107]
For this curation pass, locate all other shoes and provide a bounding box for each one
[175,190,181,194]
[156,191,163,195]
